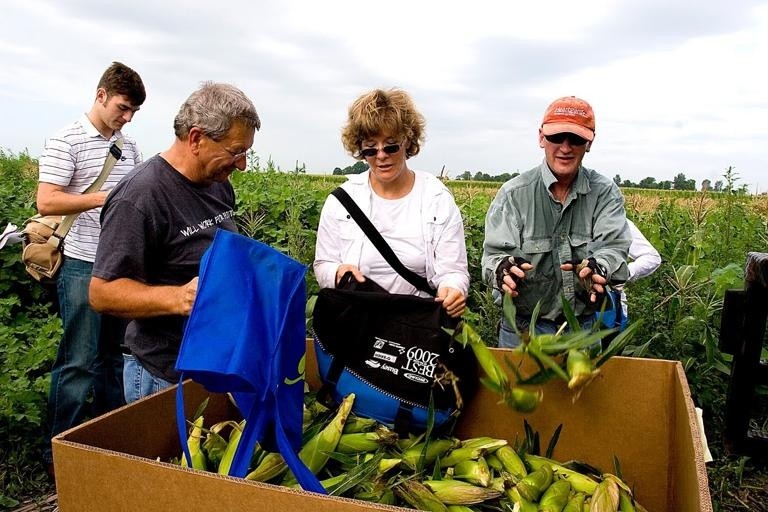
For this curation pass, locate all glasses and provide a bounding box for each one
[544,132,587,145]
[233,147,253,162]
[359,137,403,156]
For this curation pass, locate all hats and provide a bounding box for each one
[541,96,595,141]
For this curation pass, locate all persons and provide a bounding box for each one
[481,95,632,354]
[314,86,471,338]
[36,62,147,477]
[595,217,662,356]
[89,81,261,404]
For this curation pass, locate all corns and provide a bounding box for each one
[180,323,651,512]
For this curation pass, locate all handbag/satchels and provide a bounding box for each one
[19,213,63,282]
[174,228,328,495]
[311,270,476,439]
